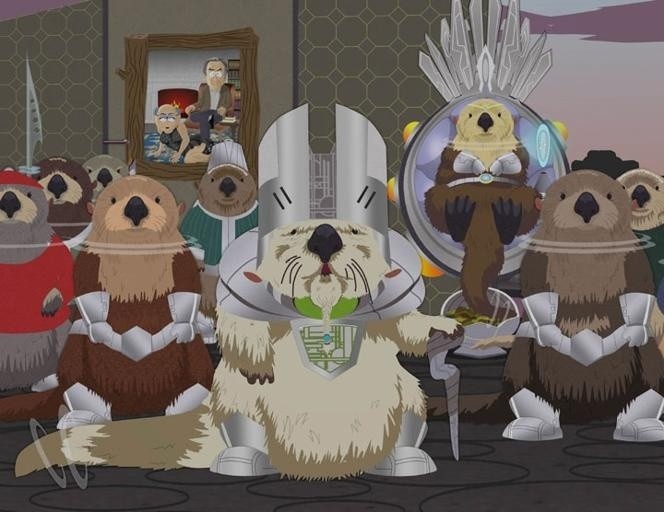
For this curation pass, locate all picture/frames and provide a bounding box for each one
[113,24,262,184]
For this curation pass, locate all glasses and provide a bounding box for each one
[156,114,177,123]
